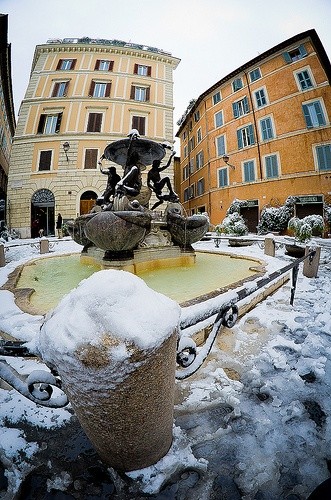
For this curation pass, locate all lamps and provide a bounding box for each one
[63,143,71,161]
[222,154,235,169]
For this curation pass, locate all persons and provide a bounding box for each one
[147,150,180,210]
[99,165,121,211]
[32,218,40,239]
[56,213,64,239]
[115,155,142,199]
